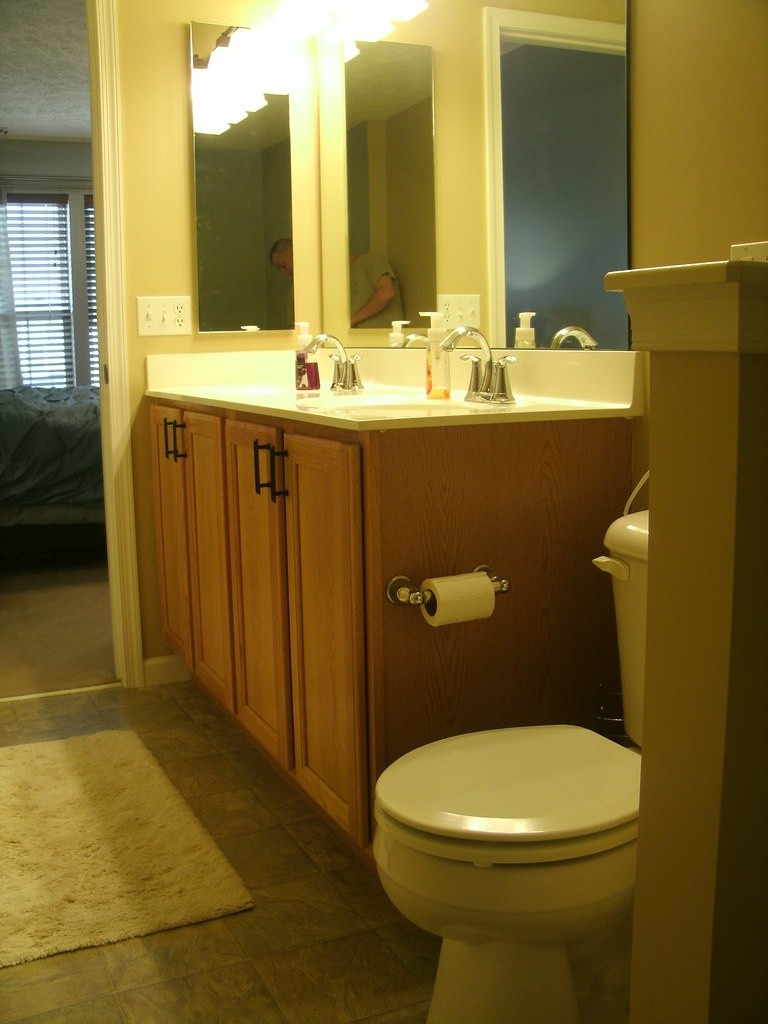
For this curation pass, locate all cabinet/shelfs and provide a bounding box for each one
[146,399,645,853]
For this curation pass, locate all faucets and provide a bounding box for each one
[549,326,599,350]
[401,333,428,347]
[438,325,494,394]
[304,333,348,388]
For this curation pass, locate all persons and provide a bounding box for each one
[270,239,403,327]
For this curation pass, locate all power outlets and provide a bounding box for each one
[137,296,193,336]
[438,294,480,329]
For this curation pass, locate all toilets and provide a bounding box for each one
[371,508,651,1024]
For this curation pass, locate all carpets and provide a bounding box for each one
[0,730,254,968]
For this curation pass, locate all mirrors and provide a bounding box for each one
[189,23,298,335]
[342,40,436,329]
[320,0,634,351]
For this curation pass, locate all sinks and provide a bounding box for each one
[335,404,468,410]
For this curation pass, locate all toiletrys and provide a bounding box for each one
[514,311,536,348]
[418,311,453,399]
[292,321,321,390]
[389,320,411,348]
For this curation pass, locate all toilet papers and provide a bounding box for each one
[420,571,495,627]
